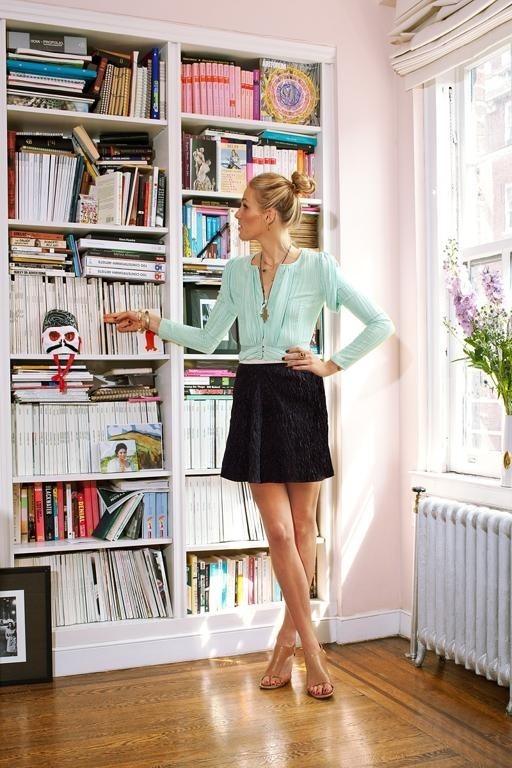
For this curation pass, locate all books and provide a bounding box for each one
[183,196,319,277]
[183,356,236,470]
[12,478,175,627]
[8,26,168,227]
[10,363,166,477]
[7,230,166,355]
[185,474,285,616]
[180,53,318,200]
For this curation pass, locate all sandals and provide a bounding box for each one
[259,641,296,690]
[304,643,335,700]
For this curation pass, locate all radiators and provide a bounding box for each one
[413,491,512,714]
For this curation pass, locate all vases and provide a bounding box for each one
[498,415,511,486]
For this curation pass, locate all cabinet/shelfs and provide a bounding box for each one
[0,0,342,681]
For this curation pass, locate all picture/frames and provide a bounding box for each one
[0,564,56,687]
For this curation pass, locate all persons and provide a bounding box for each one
[103,169,398,703]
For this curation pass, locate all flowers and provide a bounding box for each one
[436,238,512,415]
